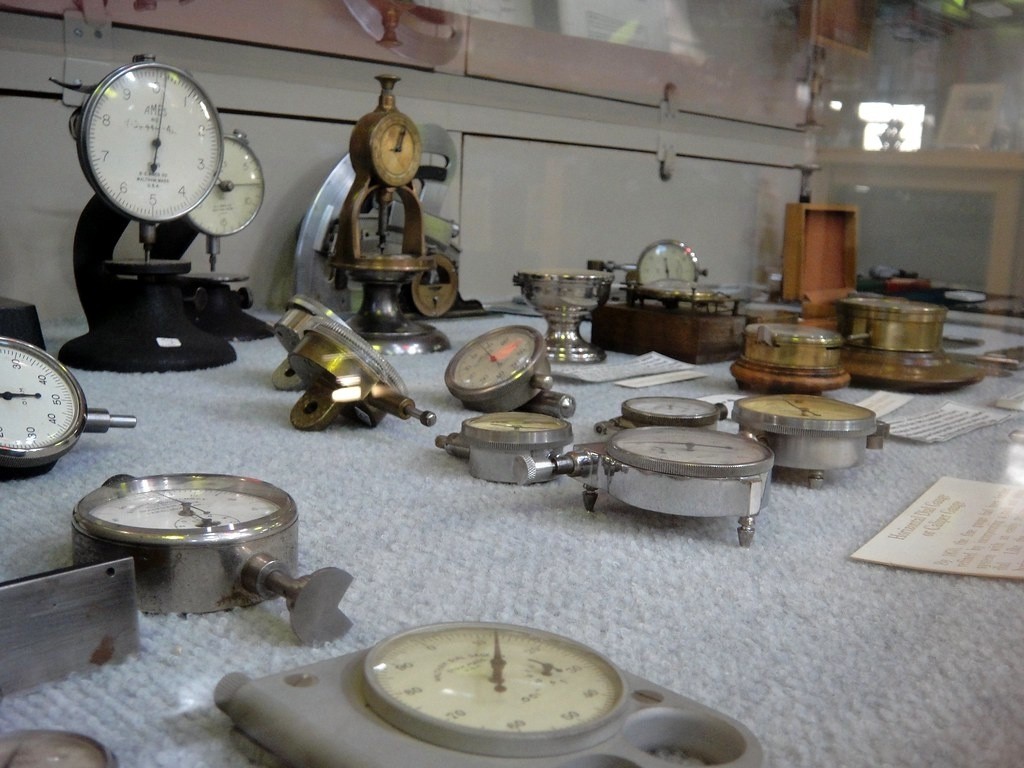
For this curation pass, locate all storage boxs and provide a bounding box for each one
[780,202,906,321]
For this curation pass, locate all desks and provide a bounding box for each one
[0,308,1024,768]
[818,149,1024,302]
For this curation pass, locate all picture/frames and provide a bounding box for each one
[936,81,1005,149]
